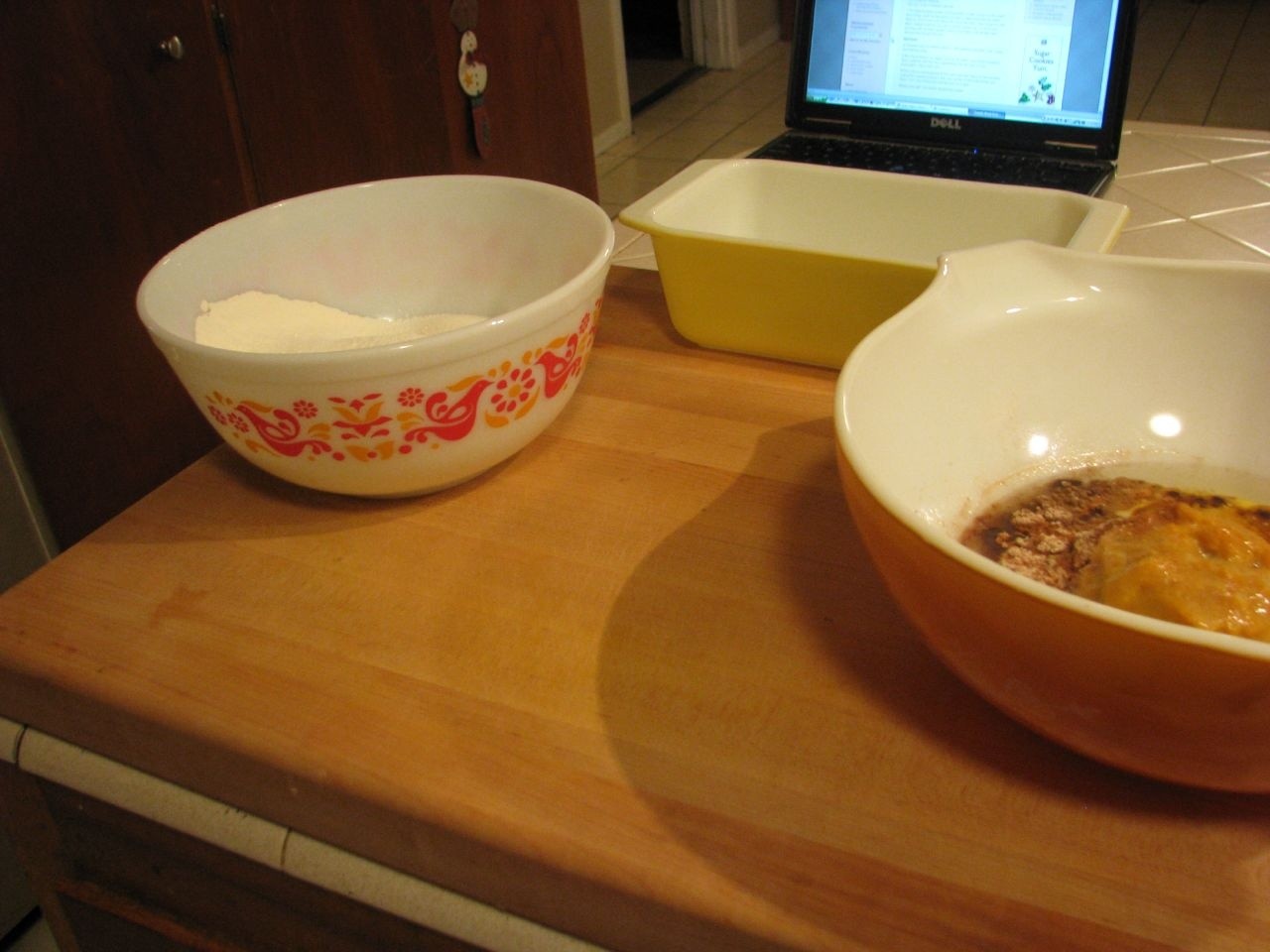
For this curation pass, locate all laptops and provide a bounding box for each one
[741,0,1138,198]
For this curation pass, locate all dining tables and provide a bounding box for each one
[0,261,1270,952]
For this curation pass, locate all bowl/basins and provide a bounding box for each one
[616,157,1133,371]
[831,240,1269,795]
[134,173,619,500]
